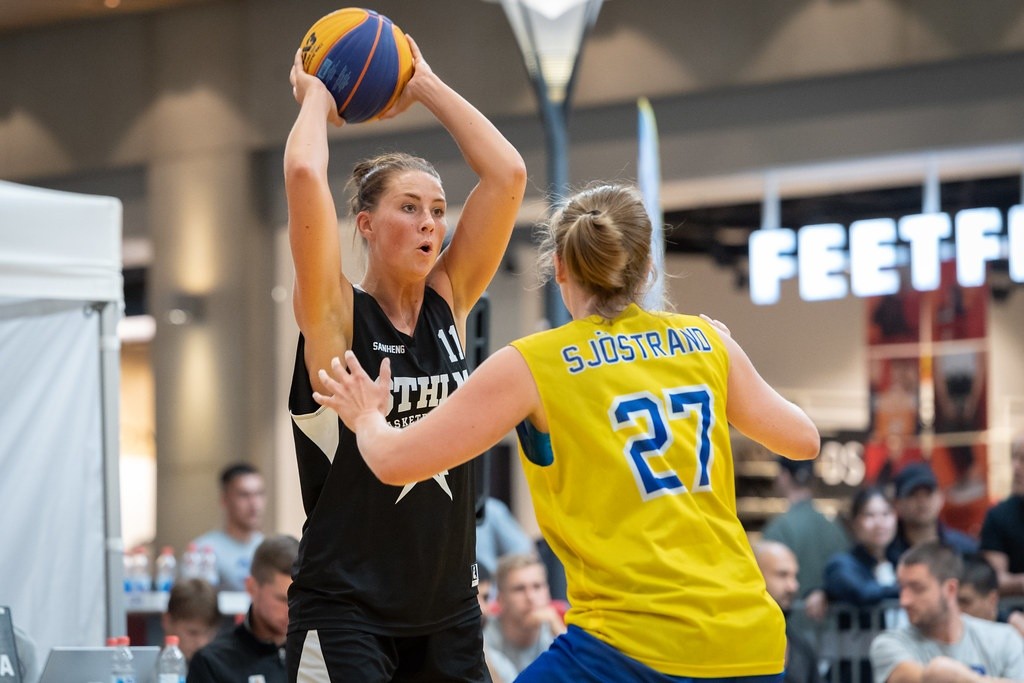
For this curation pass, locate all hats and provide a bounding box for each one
[893,461,937,497]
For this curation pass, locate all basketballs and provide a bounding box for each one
[297,6,417,125]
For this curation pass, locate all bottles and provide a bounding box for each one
[104,636,136,683]
[154,635,188,683]
[122,543,221,595]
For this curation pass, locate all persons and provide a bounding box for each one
[752,541,821,683]
[823,461,979,682]
[764,453,849,605]
[184,534,301,683]
[192,462,269,592]
[162,579,220,655]
[871,542,1024,683]
[956,552,1024,633]
[979,438,1024,593]
[283,34,527,683]
[471,498,568,683]
[313,186,821,683]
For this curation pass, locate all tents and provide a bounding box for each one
[1,183,126,655]
